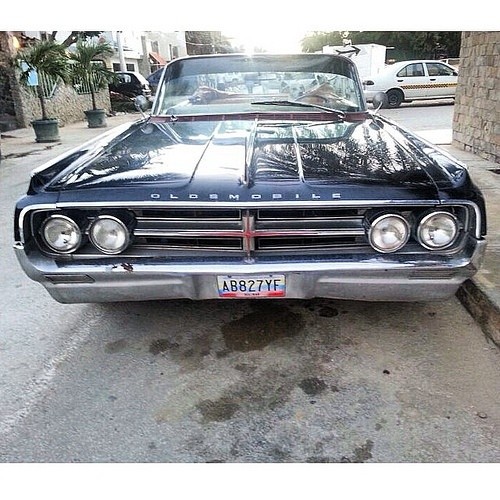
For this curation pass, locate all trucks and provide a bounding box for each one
[109,71,151,98]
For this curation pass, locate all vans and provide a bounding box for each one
[146,67,199,95]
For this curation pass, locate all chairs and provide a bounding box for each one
[413,70,422,75]
[302,83,340,105]
[116,77,125,84]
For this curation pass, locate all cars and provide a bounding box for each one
[280,72,320,94]
[12,50,487,303]
[260,73,282,89]
[362,60,458,107]
[216,72,244,86]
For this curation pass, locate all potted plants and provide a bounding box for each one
[8,36,78,142]
[66,34,126,129]
